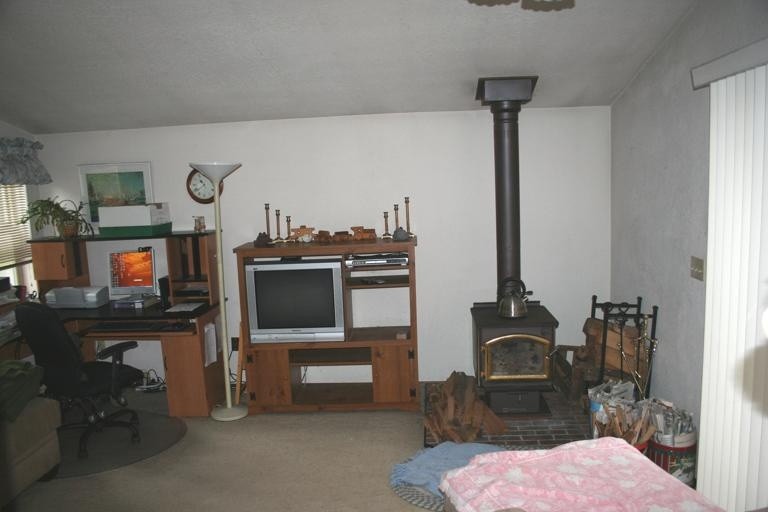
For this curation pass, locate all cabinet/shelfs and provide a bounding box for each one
[233,237,422,413]
[28,228,224,416]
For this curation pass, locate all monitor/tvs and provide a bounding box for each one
[109,248,157,302]
[245,262,344,344]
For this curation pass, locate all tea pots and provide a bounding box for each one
[495,279,534,320]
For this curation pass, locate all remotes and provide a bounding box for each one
[396,330,408,339]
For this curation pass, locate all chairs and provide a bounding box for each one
[16,301,143,457]
[556,295,659,406]
[1,360,63,509]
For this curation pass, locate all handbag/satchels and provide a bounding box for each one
[0,360,44,423]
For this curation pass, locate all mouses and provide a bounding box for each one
[172,322,185,330]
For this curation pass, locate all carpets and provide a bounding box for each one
[392,446,557,510]
[39,407,187,478]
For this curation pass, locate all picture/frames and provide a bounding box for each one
[78,161,155,230]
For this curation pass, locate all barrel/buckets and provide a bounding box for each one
[589,399,696,484]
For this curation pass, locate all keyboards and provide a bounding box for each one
[87,322,168,333]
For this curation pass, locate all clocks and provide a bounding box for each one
[186,170,224,204]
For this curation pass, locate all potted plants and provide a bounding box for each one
[23,197,93,236]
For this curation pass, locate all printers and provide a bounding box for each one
[45,286,109,309]
